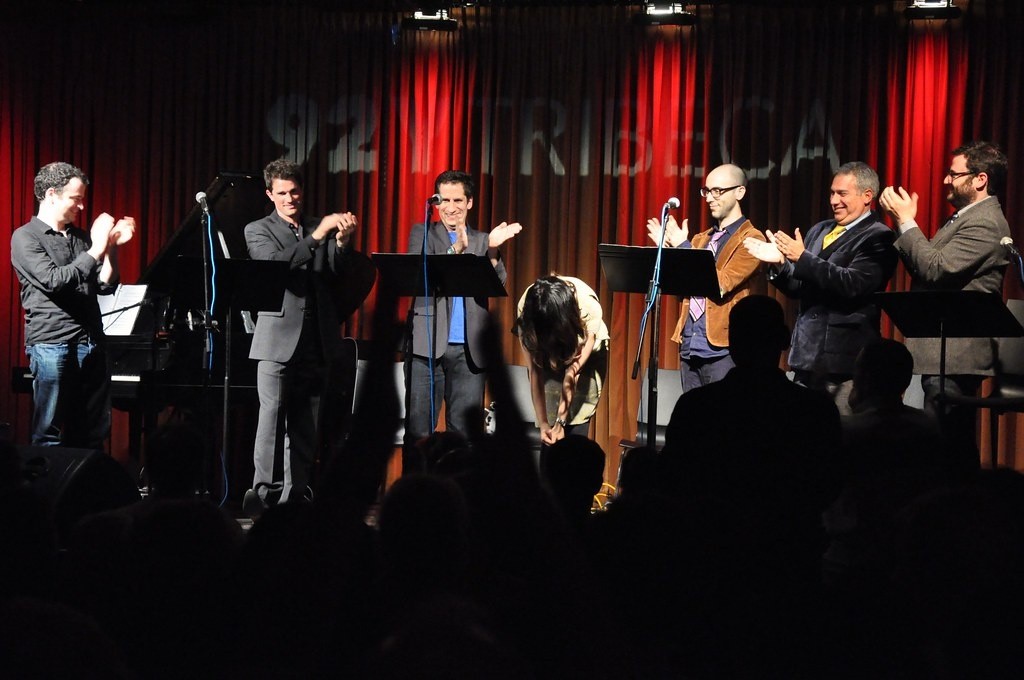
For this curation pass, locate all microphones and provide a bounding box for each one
[426,194,443,205]
[665,198,680,208]
[999,236,1022,257]
[196,192,210,217]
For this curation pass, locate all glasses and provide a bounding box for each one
[947,166,983,181]
[700,186,741,199]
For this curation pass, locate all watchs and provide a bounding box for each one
[555,418,566,427]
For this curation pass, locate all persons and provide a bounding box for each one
[0,293,1024,680]
[11,162,135,450]
[879,139,1010,443]
[512,276,609,449]
[405,170,522,454]
[744,162,895,399]
[647,164,765,392]
[244,161,358,522]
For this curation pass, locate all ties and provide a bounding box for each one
[822,223,845,250]
[688,228,728,321]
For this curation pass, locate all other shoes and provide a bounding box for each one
[243,490,269,521]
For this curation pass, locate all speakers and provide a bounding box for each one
[11,444,123,513]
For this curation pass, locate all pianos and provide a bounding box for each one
[11,172,372,495]
